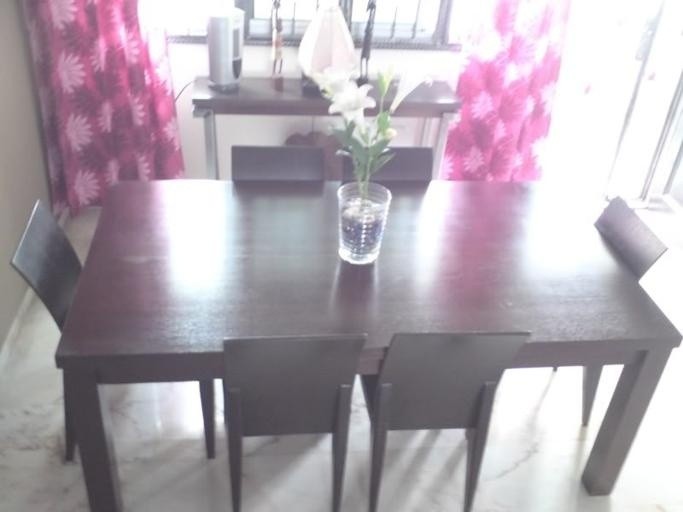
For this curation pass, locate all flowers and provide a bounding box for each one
[302,49,469,226]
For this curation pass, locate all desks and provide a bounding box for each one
[193,77,463,180]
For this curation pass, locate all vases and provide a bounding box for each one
[337,181,392,265]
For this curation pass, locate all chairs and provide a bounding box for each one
[357,331,527,509]
[221,332,368,510]
[342,148,435,181]
[553,195,668,425]
[230,146,325,180]
[10,198,82,462]
